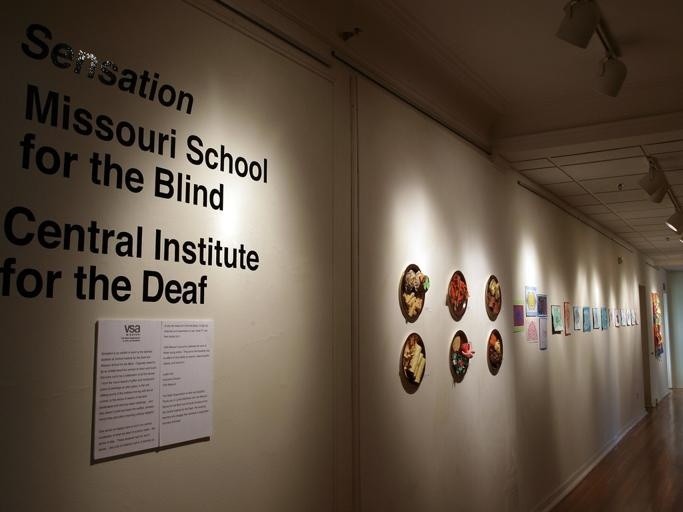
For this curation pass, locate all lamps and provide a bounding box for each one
[637,157,682,232]
[556,1,627,97]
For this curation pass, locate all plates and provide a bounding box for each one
[487,329,503,375]
[399,263,425,322]
[449,330,469,383]
[448,270,468,321]
[400,333,426,394]
[485,275,502,321]
